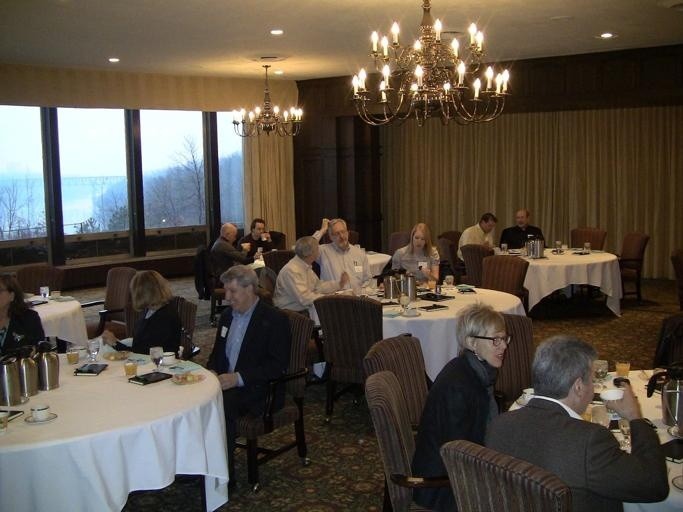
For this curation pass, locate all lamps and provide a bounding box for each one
[230,64,306,139]
[350,1,512,127]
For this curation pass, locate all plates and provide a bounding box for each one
[382,306,404,317]
[24,413,57,424]
[401,311,421,317]
[672,474,683,491]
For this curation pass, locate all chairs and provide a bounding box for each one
[604,230,651,302]
[438,438,574,511]
[570,228,608,298]
[362,336,437,439]
[459,243,495,288]
[318,228,361,246]
[312,293,383,427]
[234,309,316,495]
[439,230,462,283]
[264,231,286,249]
[364,370,450,511]
[387,231,413,256]
[14,265,63,296]
[207,267,236,327]
[479,254,530,318]
[81,267,137,336]
[493,313,535,410]
[178,297,201,360]
[261,249,298,275]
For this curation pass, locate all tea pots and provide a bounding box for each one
[648,368,683,425]
[530,240,545,258]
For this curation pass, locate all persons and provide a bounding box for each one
[413,303,506,512]
[101,270,182,355]
[311,219,372,290]
[237,218,272,253]
[0,274,45,356]
[500,209,546,250]
[485,334,670,512]
[175,265,292,507]
[210,223,262,287]
[391,223,440,286]
[457,213,498,268]
[273,236,350,317]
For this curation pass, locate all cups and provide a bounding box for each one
[31,406,50,420]
[0,412,9,432]
[406,305,417,315]
[616,361,631,379]
[593,360,609,378]
[163,352,175,363]
[600,387,624,420]
[445,276,453,293]
[67,349,79,364]
[395,273,417,302]
[592,406,609,427]
[150,347,163,372]
[501,244,508,254]
[124,360,137,378]
[580,403,592,421]
[40,287,49,301]
[618,419,630,448]
[384,276,397,299]
[86,338,99,363]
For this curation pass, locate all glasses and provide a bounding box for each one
[472,335,511,346]
